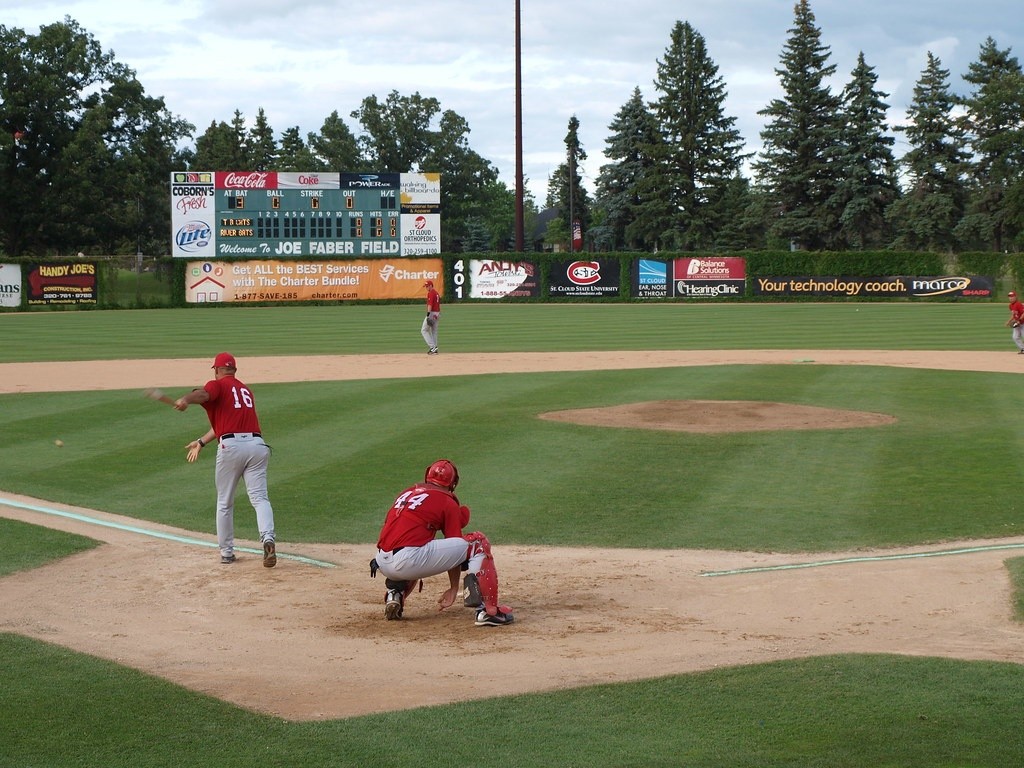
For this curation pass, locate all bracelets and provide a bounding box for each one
[197,439,205,447]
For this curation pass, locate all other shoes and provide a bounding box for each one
[1018,349,1024,354]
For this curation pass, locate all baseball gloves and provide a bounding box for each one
[463,572,483,607]
[427,317,435,326]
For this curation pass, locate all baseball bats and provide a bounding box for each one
[144,387,186,411]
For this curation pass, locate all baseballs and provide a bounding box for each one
[57,441,64,447]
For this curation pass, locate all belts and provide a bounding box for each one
[221,433,263,439]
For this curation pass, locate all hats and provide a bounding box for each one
[422,281,433,287]
[425,461,458,488]
[211,351,236,369]
[1007,291,1017,297]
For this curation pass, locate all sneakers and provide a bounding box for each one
[427,347,438,355]
[221,554,235,564]
[474,608,515,627]
[262,537,277,568]
[383,589,405,621]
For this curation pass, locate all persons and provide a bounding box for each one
[421,280,440,354]
[1005,291,1024,354]
[173,352,277,567]
[376,460,514,626]
